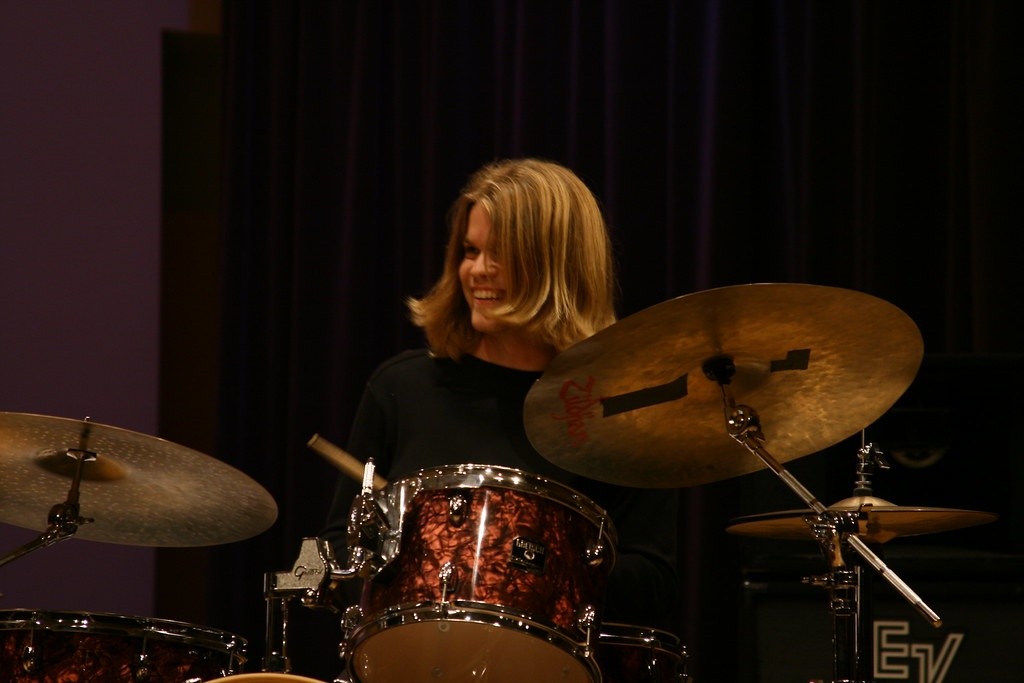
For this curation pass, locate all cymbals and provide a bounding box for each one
[523,282,927,490]
[724,495,1001,546]
[0,411,280,548]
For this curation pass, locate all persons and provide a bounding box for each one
[318,159,680,620]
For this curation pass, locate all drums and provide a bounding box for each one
[0,606,249,683]
[181,671,334,683]
[588,618,690,683]
[340,463,621,683]
